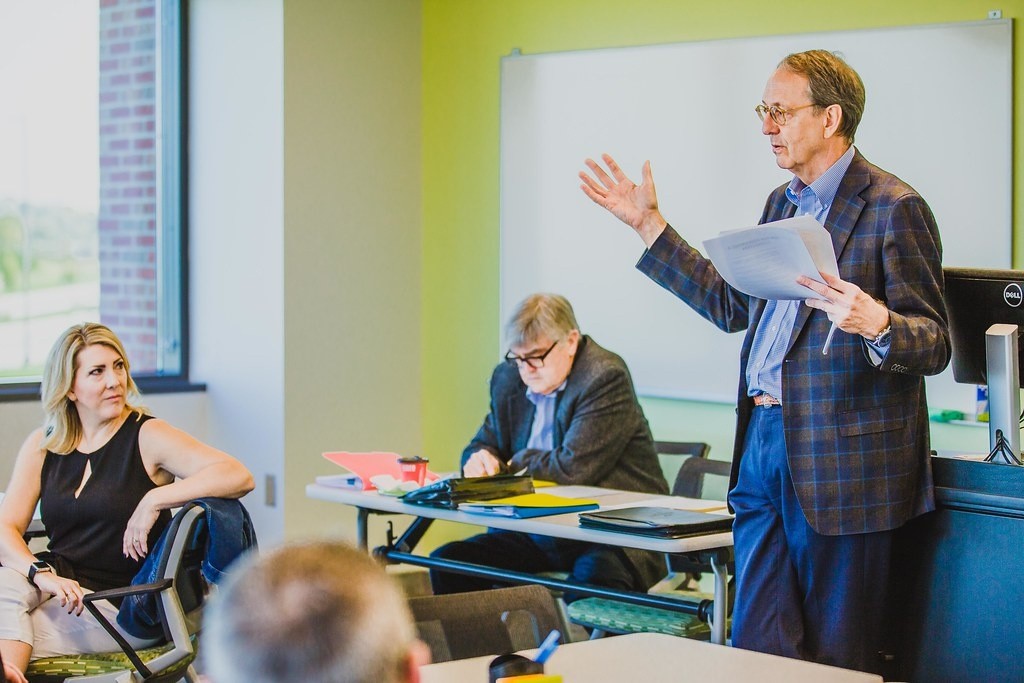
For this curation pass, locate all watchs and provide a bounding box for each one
[28,561,51,586]
[865,322,892,349]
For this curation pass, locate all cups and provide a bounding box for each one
[398,455,429,491]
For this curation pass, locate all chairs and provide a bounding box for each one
[490,441,733,641]
[391,572,570,663]
[26,497,258,683]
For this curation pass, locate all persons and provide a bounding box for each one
[579,50,952,673]
[0,321,254,683]
[201,538,430,683]
[429,291,675,660]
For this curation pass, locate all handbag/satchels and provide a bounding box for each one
[403,474,537,510]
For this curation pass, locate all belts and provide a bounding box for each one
[754,394,780,407]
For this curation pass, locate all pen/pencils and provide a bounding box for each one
[822,322,838,355]
[532,629,561,664]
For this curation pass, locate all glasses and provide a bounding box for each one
[504,337,559,368]
[755,104,829,126]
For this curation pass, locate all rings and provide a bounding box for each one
[133,539,139,544]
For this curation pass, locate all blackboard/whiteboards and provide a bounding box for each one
[495,17,1015,430]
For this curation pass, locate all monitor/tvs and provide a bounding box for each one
[941,267,1024,468]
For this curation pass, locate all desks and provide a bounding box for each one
[304,477,735,646]
[416,632,885,683]
